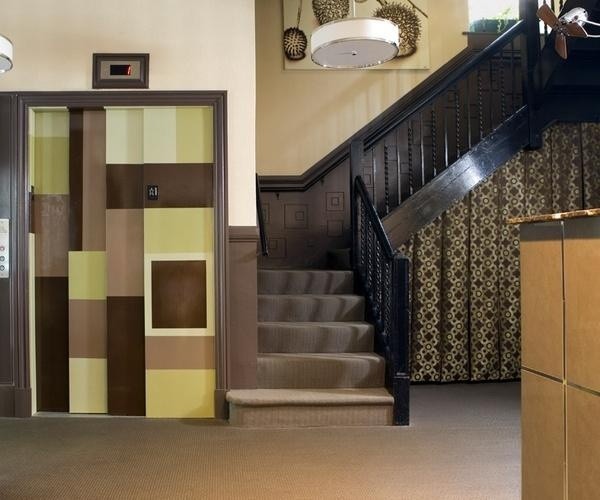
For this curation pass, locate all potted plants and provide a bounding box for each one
[469,7,517,32]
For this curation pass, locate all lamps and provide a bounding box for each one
[310,0,399,69]
[0,33,13,73]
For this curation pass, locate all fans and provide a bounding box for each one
[536,4,600,59]
